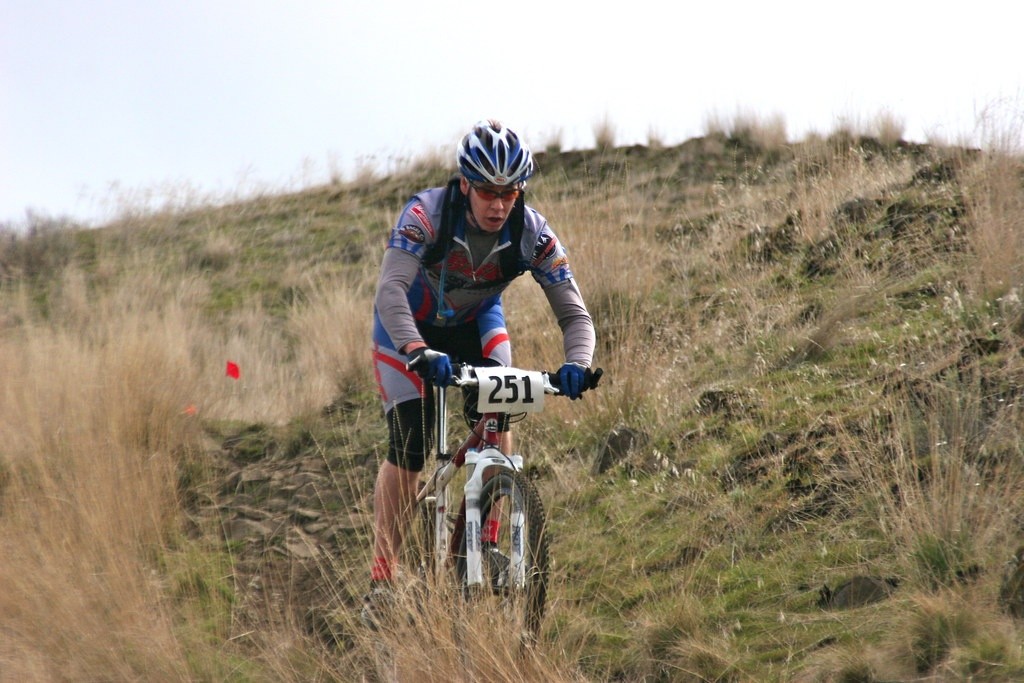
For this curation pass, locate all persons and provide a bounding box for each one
[362,120,597,625]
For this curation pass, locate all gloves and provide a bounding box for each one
[556,365,589,401]
[407,347,461,388]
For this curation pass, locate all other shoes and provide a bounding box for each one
[360,588,388,620]
[484,549,508,579]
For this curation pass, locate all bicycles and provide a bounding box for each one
[405,353,603,660]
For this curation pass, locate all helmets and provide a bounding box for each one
[457,118,533,186]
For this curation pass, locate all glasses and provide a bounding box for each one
[467,178,524,200]
